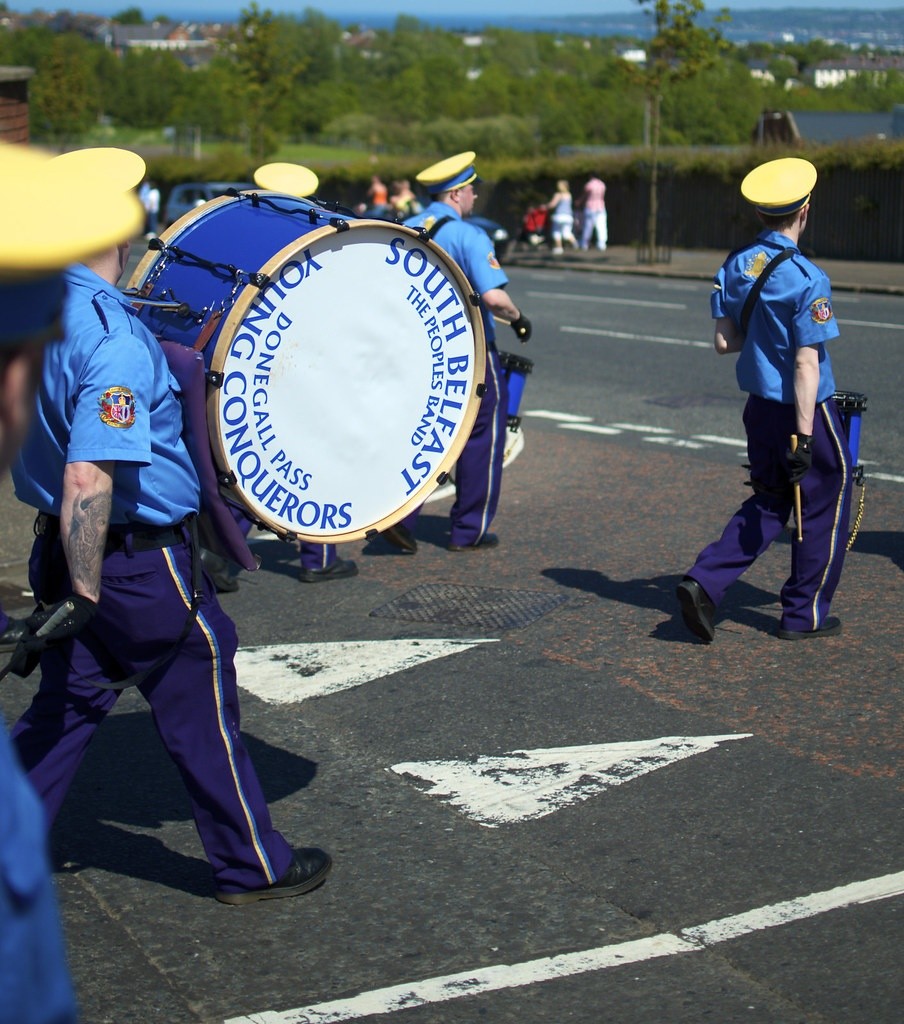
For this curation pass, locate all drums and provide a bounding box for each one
[129,188,488,548]
[829,390,869,485]
[495,350,534,432]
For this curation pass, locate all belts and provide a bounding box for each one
[37,512,183,552]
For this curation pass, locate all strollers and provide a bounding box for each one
[514,208,553,252]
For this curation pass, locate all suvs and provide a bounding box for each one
[164,182,256,230]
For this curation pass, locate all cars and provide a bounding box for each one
[364,203,508,258]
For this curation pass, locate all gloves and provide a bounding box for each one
[786,432,812,482]
[510,310,532,343]
[17,594,99,653]
[0,615,27,651]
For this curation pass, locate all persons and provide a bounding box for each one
[673,157,853,641]
[385,150,532,553]
[354,174,415,217]
[0,146,330,1024]
[198,162,358,591]
[519,171,608,254]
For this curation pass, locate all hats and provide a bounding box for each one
[0,143,146,345]
[415,151,484,194]
[253,162,319,197]
[741,157,817,217]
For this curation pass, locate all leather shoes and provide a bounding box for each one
[778,616,842,640]
[215,846,332,904]
[448,534,499,551]
[199,547,239,592]
[676,579,716,641]
[381,523,418,554]
[298,557,358,582]
